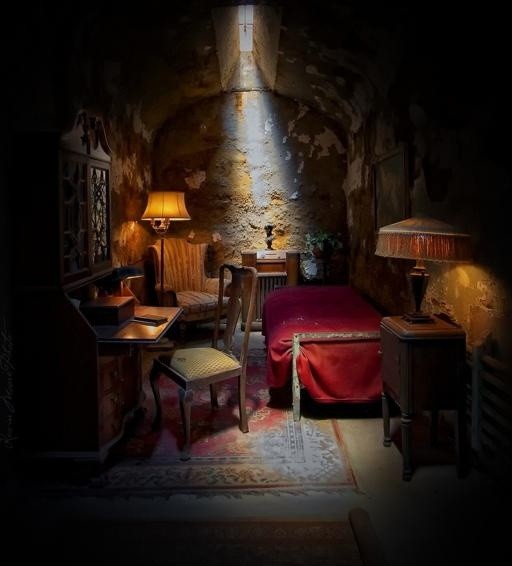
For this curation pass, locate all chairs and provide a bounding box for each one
[146,237,243,346]
[148,263,259,462]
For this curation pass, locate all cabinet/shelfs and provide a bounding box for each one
[0,108,183,466]
[378,313,466,482]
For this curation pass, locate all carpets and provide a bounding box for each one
[23,347,366,498]
[99,507,387,566]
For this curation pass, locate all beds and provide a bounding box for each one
[261,285,385,422]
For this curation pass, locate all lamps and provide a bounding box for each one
[140,191,191,309]
[372,215,476,325]
